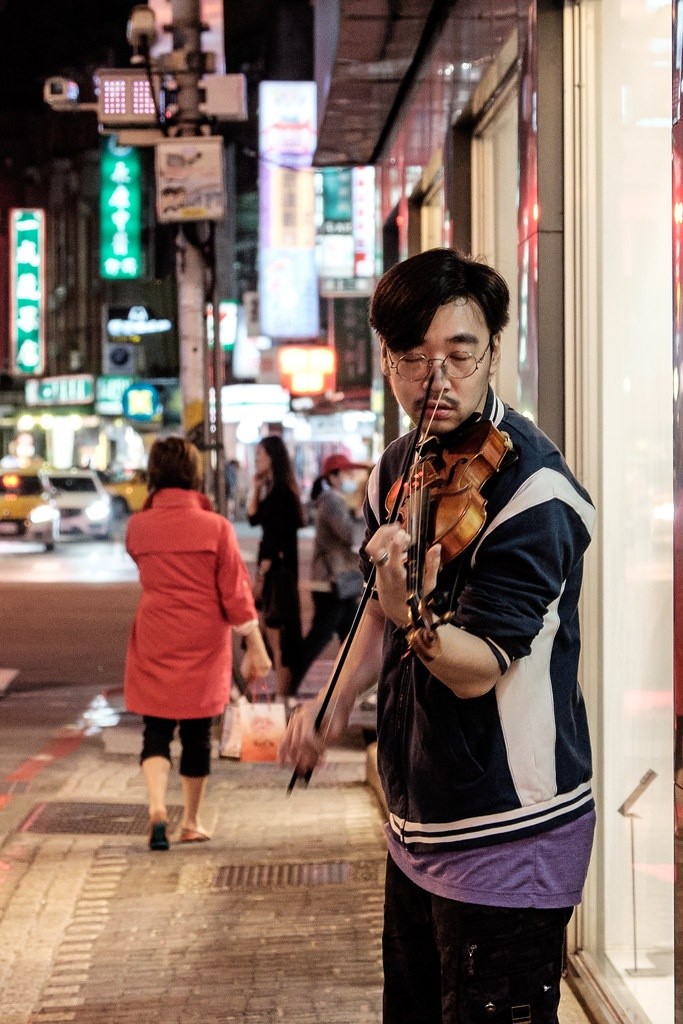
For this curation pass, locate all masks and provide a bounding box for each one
[342,481,356,492]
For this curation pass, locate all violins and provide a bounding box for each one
[385,419,509,662]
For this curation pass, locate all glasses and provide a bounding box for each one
[384,341,492,382]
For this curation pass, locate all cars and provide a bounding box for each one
[0,468,150,551]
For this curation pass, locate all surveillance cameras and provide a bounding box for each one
[42,77,80,103]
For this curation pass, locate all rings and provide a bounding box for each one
[377,552,390,568]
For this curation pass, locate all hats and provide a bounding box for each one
[324,455,367,475]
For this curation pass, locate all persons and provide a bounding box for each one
[224,460,241,521]
[277,246,596,1024]
[245,436,304,675]
[124,437,274,850]
[286,451,372,698]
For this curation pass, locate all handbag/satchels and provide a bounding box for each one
[329,571,365,598]
[239,677,287,763]
[218,678,249,758]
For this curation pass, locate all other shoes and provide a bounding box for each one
[147,814,171,851]
[361,695,377,710]
[178,825,211,841]
